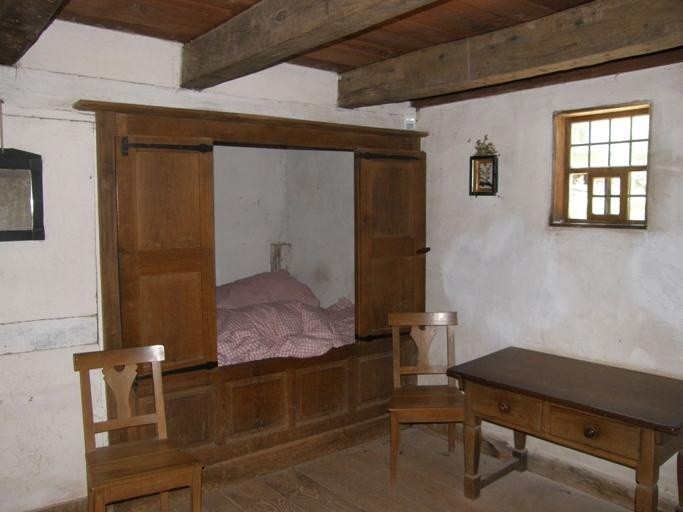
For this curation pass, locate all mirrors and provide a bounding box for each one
[0,150,45,241]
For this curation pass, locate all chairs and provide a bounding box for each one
[387,312,466,485]
[73,345,203,511]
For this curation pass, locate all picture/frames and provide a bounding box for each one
[470,155,497,195]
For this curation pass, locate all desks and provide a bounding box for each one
[446,348,683,512]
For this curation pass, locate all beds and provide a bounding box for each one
[72,98,427,484]
